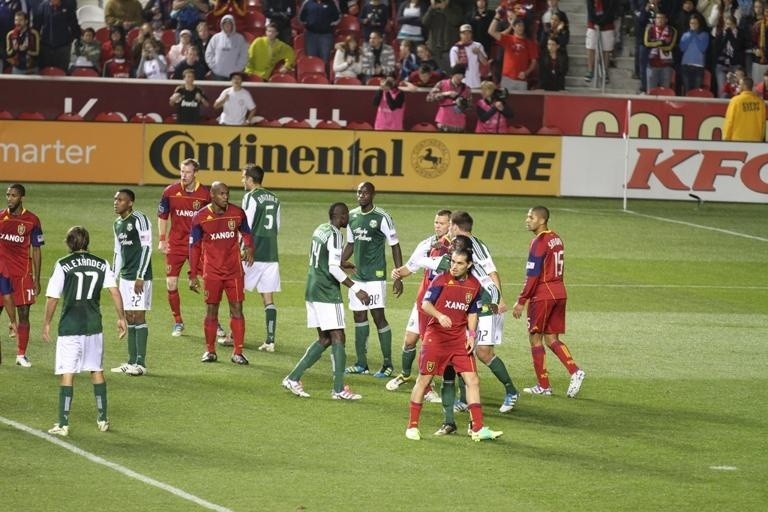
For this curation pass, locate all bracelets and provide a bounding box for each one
[158,234,166,241]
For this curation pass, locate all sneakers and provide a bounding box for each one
[47,423,69,436]
[111,363,147,376]
[171,321,275,352]
[500,392,519,414]
[346,362,470,413]
[281,376,310,398]
[230,353,250,366]
[434,423,458,436]
[523,383,554,396]
[199,351,217,364]
[16,355,30,368]
[567,370,584,399]
[96,419,110,431]
[468,425,503,441]
[332,387,362,400]
[405,426,420,440]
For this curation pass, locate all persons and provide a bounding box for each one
[109,188,153,377]
[0,275,18,338]
[280,201,372,401]
[168,68,210,124]
[2,1,767,103]
[41,225,129,438]
[0,184,44,370]
[424,63,473,133]
[187,180,255,366]
[212,71,257,126]
[338,178,519,444]
[511,205,586,399]
[156,158,212,338]
[473,79,514,134]
[216,163,282,353]
[371,77,406,132]
[721,77,766,142]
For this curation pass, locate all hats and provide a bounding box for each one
[460,24,471,33]
[451,64,467,77]
[181,29,194,36]
[228,70,248,82]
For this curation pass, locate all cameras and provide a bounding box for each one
[383,79,397,89]
